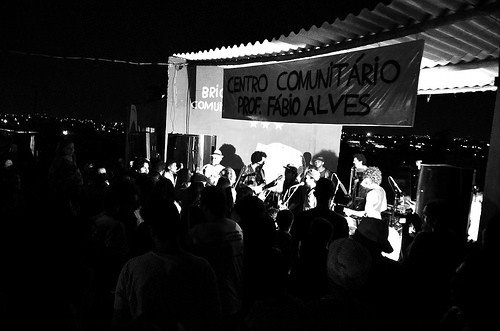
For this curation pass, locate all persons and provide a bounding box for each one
[0,121,500,331]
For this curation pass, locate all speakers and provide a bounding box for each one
[415,164,477,242]
[166,134,217,174]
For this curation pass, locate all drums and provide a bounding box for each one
[383,204,394,215]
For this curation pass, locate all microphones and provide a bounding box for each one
[334,174,347,195]
[291,181,305,188]
[389,176,402,193]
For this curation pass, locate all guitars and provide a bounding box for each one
[252,174,284,196]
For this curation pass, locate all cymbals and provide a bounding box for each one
[344,196,364,200]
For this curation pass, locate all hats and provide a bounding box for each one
[188,223,224,244]
[357,218,393,252]
